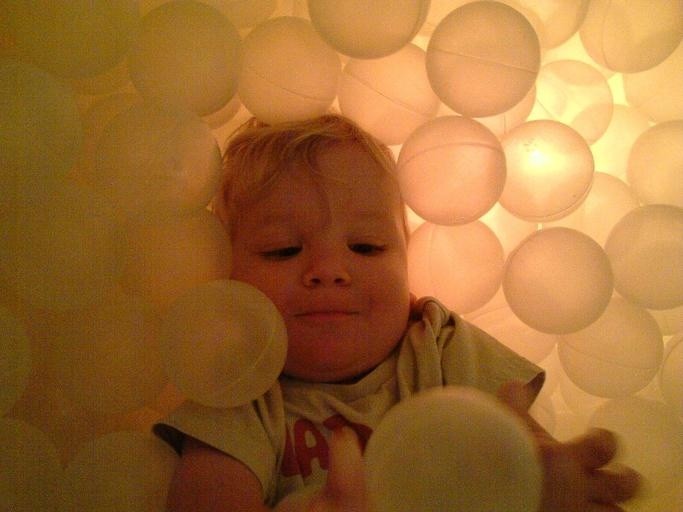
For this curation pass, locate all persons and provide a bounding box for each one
[152,115,641,512]
